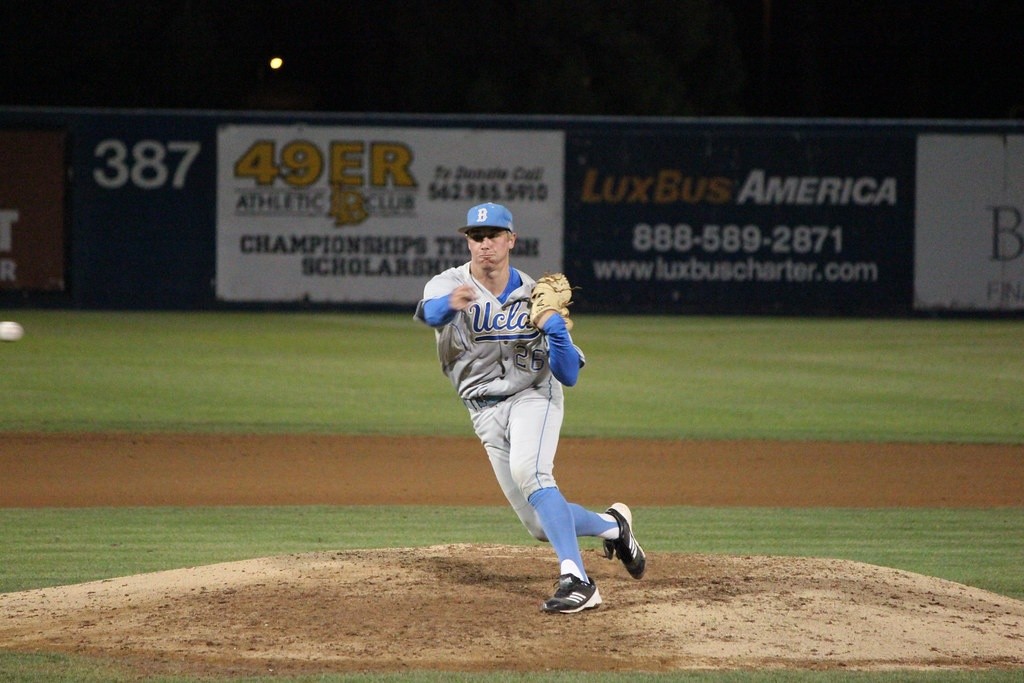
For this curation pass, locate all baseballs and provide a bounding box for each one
[0,321,24,342]
[269,57,283,70]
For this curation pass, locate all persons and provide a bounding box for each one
[411,201,649,614]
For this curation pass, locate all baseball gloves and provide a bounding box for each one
[527,269,582,331]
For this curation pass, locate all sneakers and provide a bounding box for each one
[540,574,603,616]
[603,503,648,580]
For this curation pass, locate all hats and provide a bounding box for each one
[457,203,515,233]
